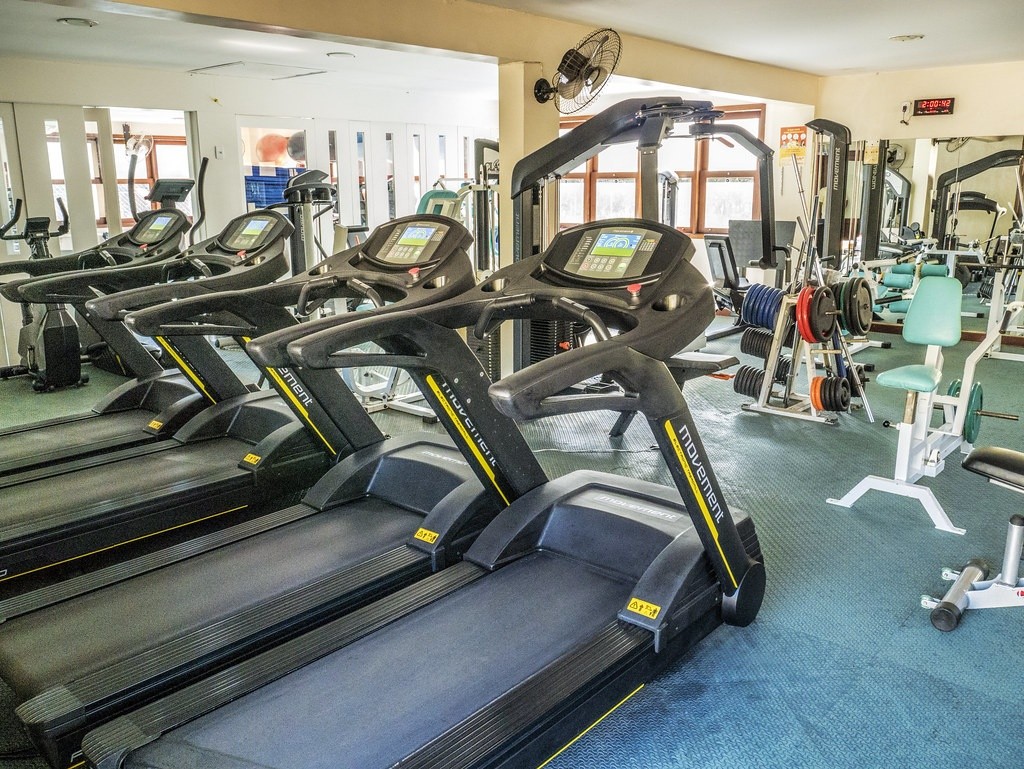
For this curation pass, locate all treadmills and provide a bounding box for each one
[81,215,768,768]
[1,213,550,768]
[1,207,208,476]
[1,209,334,584]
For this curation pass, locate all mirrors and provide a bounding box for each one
[817,136,1023,263]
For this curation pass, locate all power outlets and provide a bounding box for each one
[902,101,911,114]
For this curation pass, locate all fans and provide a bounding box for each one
[126,131,154,162]
[932,137,972,152]
[536,28,623,115]
[886,144,906,173]
